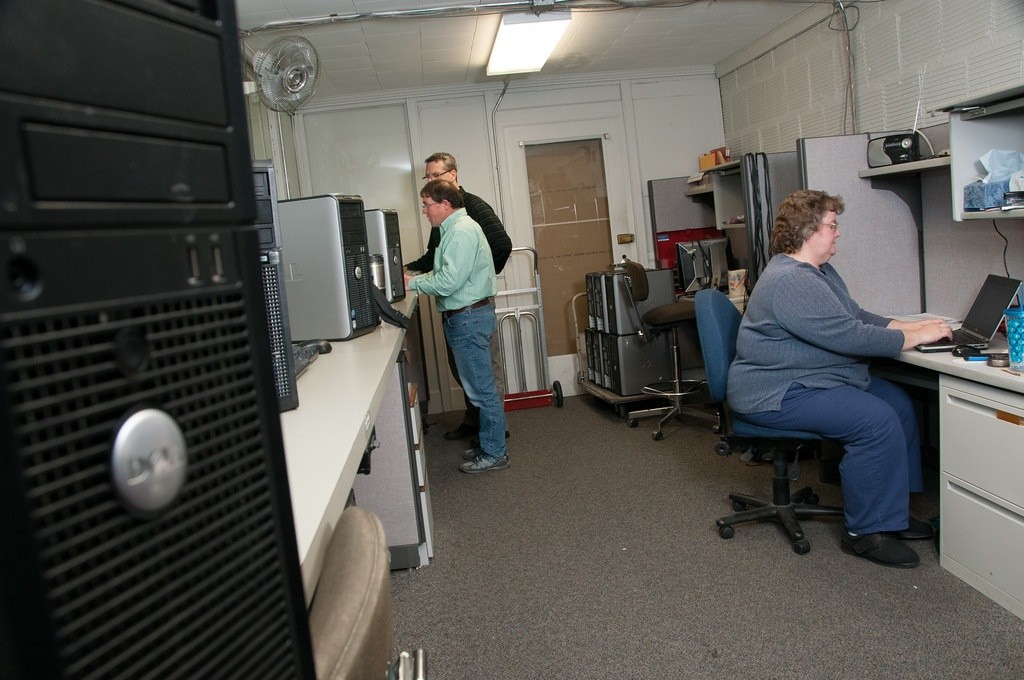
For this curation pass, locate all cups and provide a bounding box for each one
[1003,308,1024,372]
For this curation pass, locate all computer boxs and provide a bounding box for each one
[0,0,320,680]
[275,193,377,343]
[584,265,676,397]
[364,208,408,305]
[250,157,299,414]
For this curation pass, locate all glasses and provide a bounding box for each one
[421,170,450,186]
[819,222,840,232]
[421,203,439,212]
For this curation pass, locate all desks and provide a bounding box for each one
[278,288,434,617]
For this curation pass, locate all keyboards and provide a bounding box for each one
[292,343,320,381]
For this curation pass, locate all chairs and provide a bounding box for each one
[694,287,847,557]
[621,256,721,441]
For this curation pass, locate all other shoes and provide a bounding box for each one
[471,430,510,448]
[840,527,919,568]
[444,423,478,440]
[879,516,933,538]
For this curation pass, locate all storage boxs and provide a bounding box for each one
[728,268,747,299]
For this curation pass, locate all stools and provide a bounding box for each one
[307,505,428,680]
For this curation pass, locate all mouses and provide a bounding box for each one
[951,345,981,357]
[299,338,332,354]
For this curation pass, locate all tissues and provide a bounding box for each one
[964,149,1024,210]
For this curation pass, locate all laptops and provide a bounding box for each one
[913,274,1023,353]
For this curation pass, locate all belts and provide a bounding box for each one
[442,298,490,319]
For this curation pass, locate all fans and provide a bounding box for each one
[254,34,320,113]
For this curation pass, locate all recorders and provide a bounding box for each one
[868,62,928,168]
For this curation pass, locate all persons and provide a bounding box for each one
[726,188,952,570]
[402,151,512,443]
[404,180,511,474]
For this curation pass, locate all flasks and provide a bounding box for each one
[368,253,387,298]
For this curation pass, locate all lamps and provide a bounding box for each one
[486,8,572,76]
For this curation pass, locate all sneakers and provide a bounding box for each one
[460,453,510,473]
[463,447,486,459]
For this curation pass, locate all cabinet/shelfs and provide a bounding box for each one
[647,149,798,385]
[794,81,1024,622]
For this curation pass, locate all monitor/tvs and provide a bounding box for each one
[675,237,735,298]
